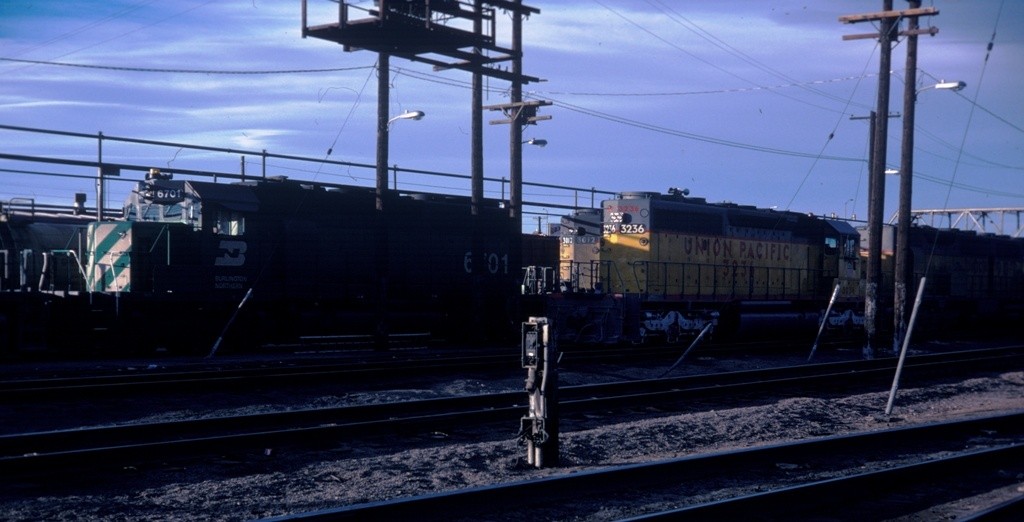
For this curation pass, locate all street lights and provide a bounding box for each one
[892,78,967,358]
[375,109,426,199]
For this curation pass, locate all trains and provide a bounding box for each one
[80,167,1024,316]
[0,191,125,293]
